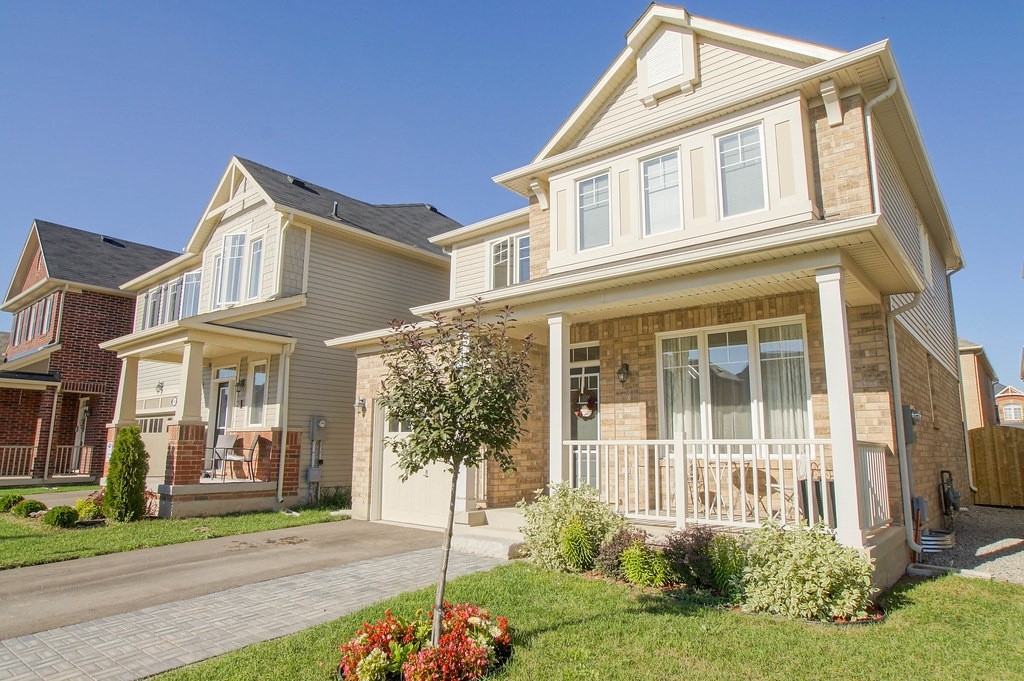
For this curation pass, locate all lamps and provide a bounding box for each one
[235,379,245,398]
[616,362,631,387]
[156,381,164,393]
[353,396,365,417]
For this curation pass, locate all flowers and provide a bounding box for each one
[572,388,596,418]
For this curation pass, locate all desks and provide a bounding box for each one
[709,460,752,521]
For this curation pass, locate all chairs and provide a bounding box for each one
[220,434,260,482]
[912,496,955,567]
[766,448,811,519]
[201,434,238,480]
[669,450,716,515]
